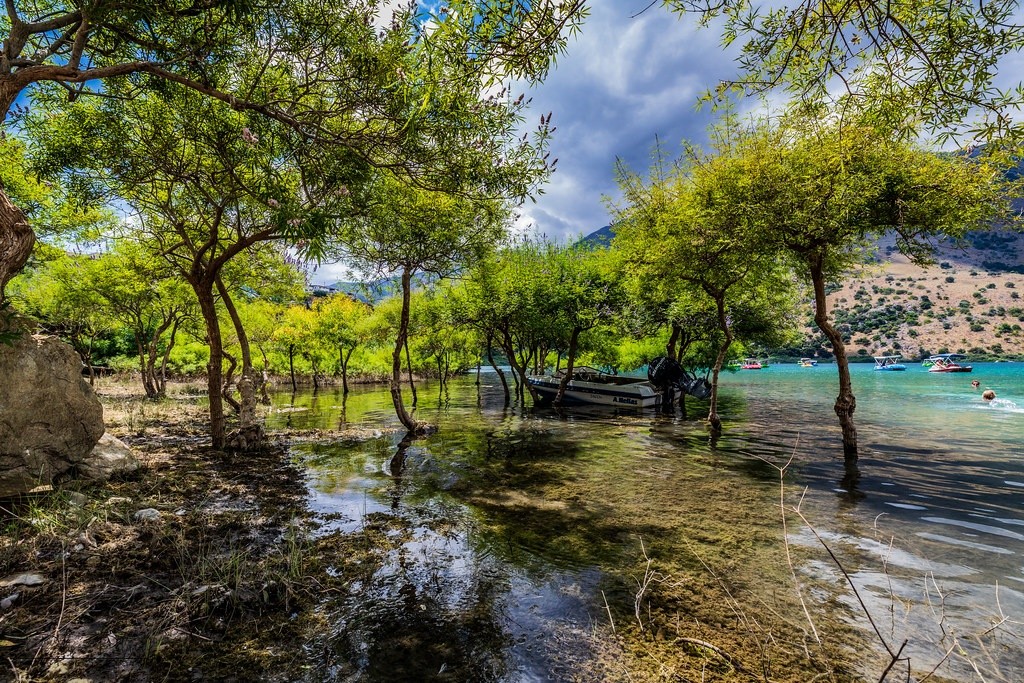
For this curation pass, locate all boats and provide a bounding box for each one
[739,357,761,369]
[524,363,682,406]
[796,357,818,366]
[927,353,970,372]
[874,355,905,371]
